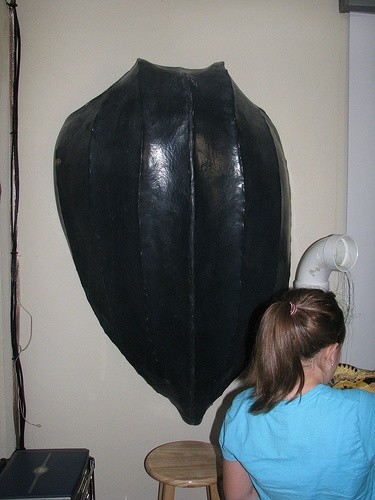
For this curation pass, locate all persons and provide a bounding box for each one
[219,288,375,500]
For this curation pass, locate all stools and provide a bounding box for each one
[144,441,223,500]
